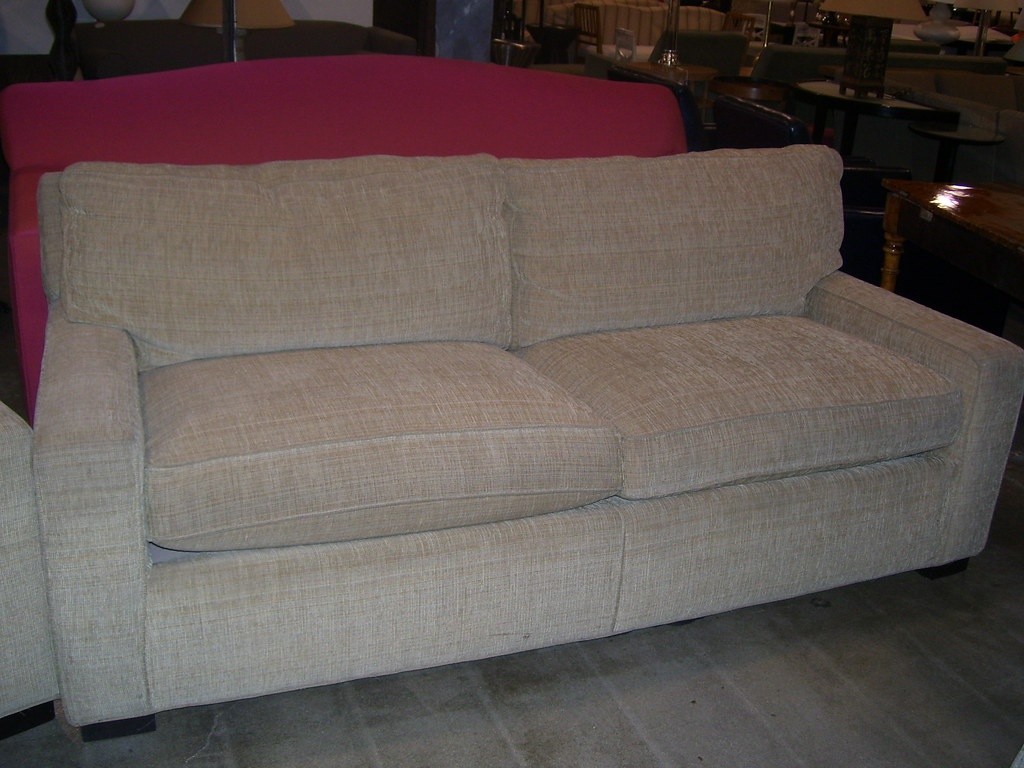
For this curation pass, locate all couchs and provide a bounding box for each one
[751,44,1007,128]
[535,0,726,44]
[647,30,749,123]
[72,20,418,80]
[0,54,688,427]
[30,144,1024,744]
[1,401,61,740]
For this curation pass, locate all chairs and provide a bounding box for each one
[711,92,1009,337]
[574,3,603,54]
[721,12,755,41]
[607,63,717,151]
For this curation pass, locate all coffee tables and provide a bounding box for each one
[585,46,752,79]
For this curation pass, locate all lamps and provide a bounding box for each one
[819,0,928,100]
[178,0,295,62]
[953,0,1019,56]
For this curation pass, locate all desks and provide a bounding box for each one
[791,77,960,165]
[879,178,1024,293]
[808,21,850,48]
[909,122,1006,182]
[631,63,717,123]
